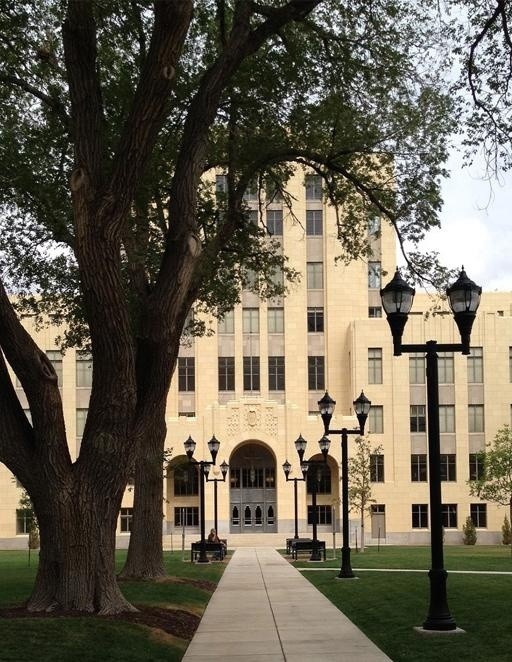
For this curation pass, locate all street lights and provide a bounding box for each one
[295,432,332,563]
[204,460,230,561]
[184,433,221,566]
[379,263,484,635]
[317,388,373,581]
[282,459,309,558]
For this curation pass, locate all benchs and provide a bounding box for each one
[190,542,222,562]
[292,541,326,561]
[286,538,311,555]
[197,539,227,555]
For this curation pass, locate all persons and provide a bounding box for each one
[208,528,226,562]
